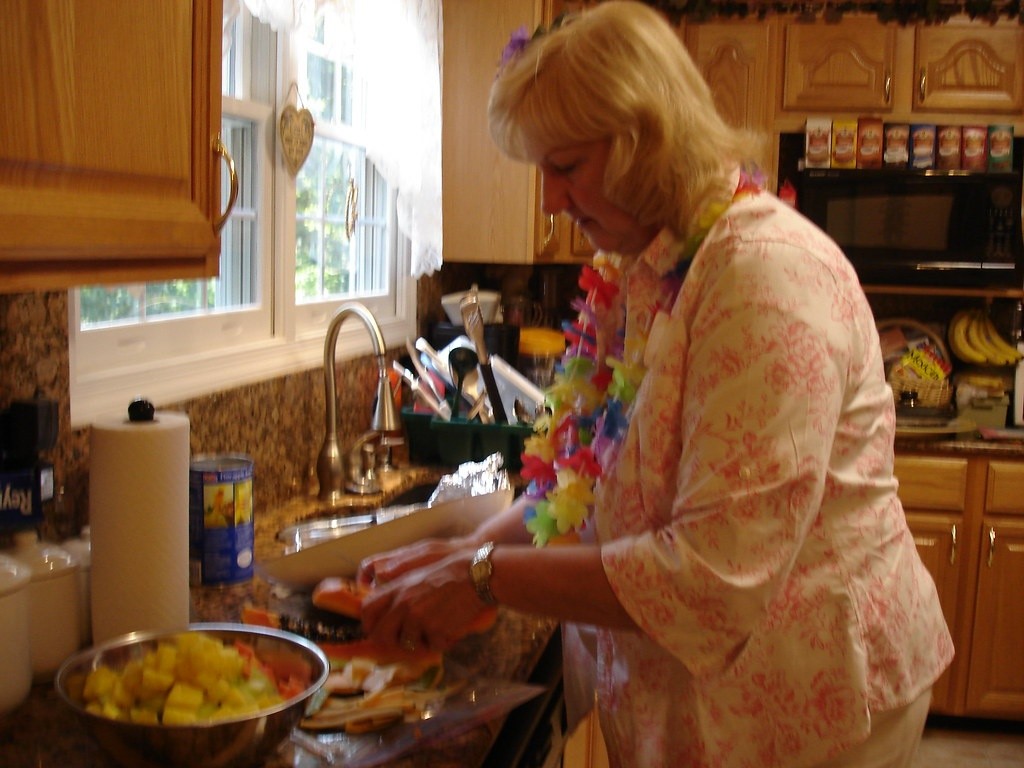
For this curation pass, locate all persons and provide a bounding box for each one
[359,0,955,767]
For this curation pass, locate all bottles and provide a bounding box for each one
[898,391,922,407]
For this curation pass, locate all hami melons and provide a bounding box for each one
[238,578,497,674]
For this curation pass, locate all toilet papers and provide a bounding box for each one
[88,409,192,650]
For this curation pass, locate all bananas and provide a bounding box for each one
[947,303,1024,367]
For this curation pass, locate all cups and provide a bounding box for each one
[10,532,79,679]
[0,554,32,714]
[62,529,90,647]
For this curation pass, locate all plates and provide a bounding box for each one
[895,419,976,439]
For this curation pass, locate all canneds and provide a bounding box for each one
[189,454,254,584]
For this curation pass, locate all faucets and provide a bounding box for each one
[315,298,399,503]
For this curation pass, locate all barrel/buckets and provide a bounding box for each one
[519,326,567,424]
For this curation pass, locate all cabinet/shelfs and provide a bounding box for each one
[778,0,1024,140]
[886,419,1024,726]
[533,0,779,267]
[0,0,239,294]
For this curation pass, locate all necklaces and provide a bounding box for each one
[504,169,762,551]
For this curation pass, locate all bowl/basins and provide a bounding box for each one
[275,515,399,554]
[896,407,955,426]
[56,623,330,768]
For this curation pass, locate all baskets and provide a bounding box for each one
[875,320,954,408]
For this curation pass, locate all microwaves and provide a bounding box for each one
[774,131,1024,289]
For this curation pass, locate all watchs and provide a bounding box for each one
[468,540,500,606]
